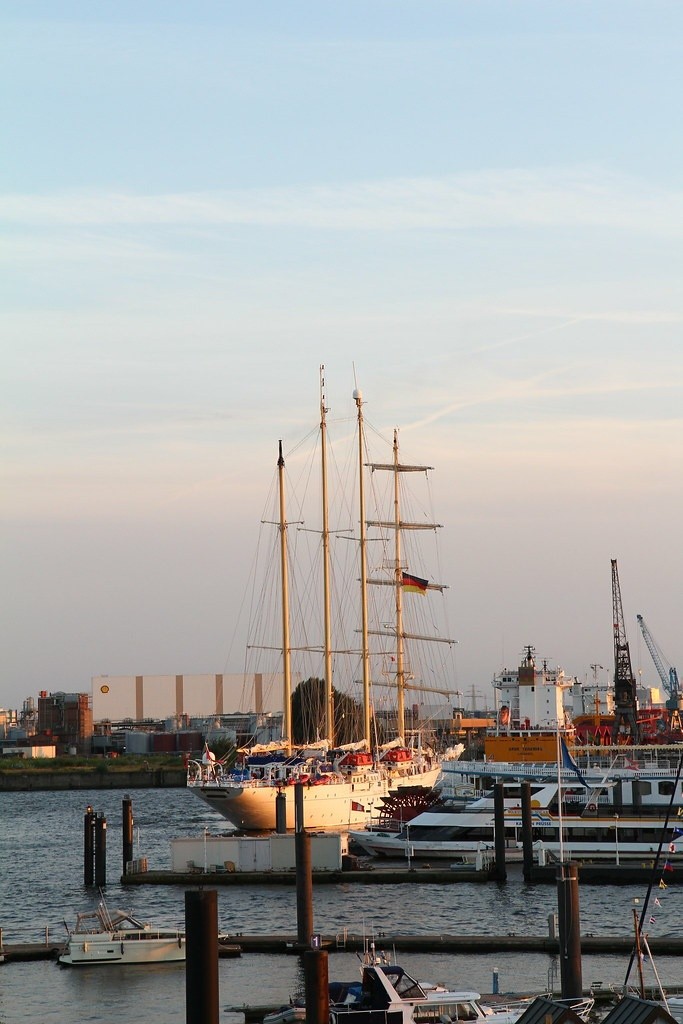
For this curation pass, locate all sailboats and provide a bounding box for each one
[185,365,466,833]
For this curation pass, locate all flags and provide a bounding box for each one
[659,879,668,890]
[352,801,364,811]
[665,861,673,871]
[670,843,675,853]
[650,915,656,926]
[641,953,647,962]
[624,757,639,770]
[675,827,683,834]
[677,807,683,816]
[655,898,662,907]
[403,572,428,596]
[560,736,591,791]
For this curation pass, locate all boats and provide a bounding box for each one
[348,553,683,864]
[263,918,594,1024]
[58,908,241,966]
